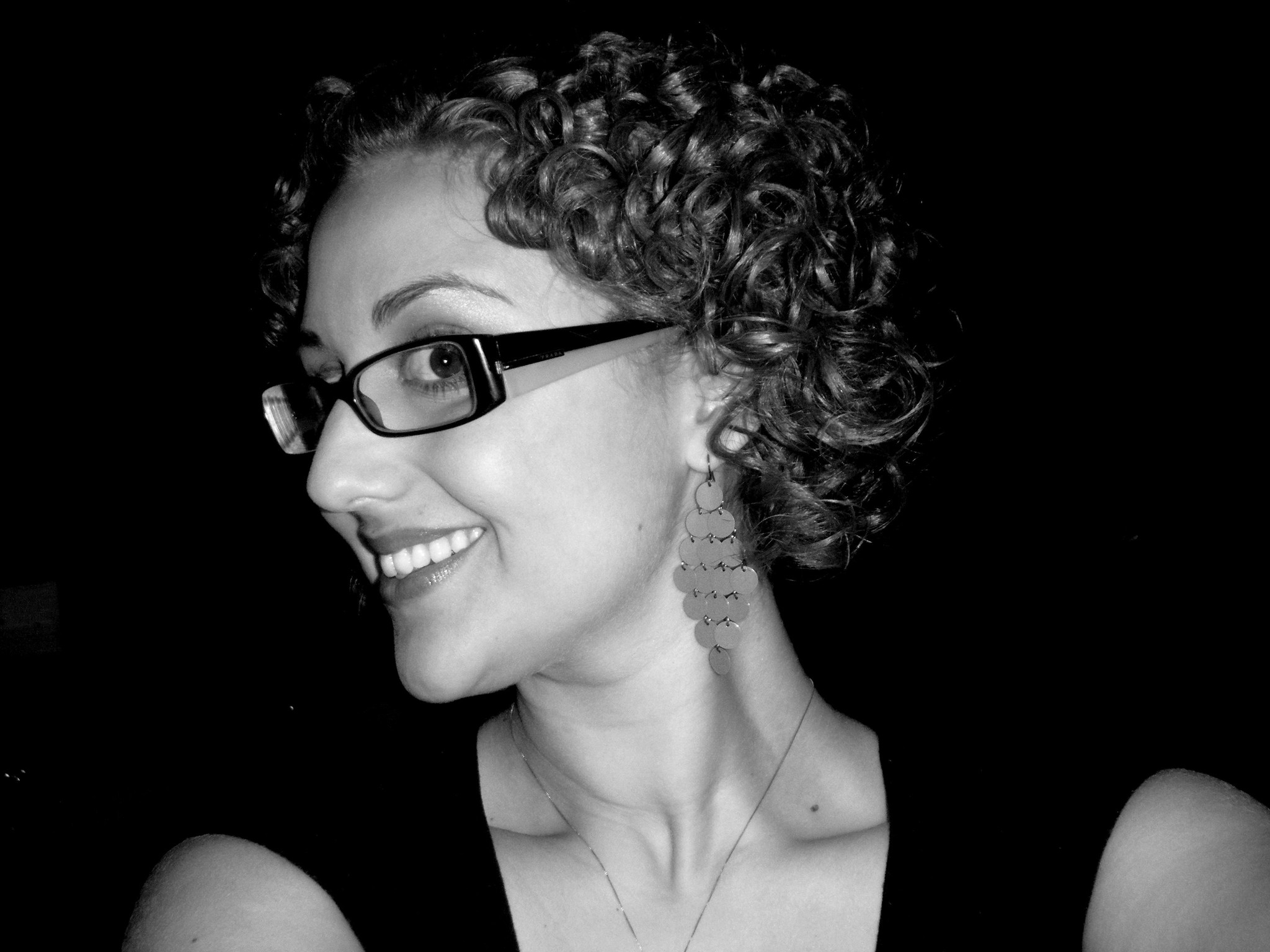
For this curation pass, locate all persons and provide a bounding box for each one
[127,33,1270,952]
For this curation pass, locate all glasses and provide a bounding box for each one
[259,317,672,457]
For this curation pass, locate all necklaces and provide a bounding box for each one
[509,678,815,952]
